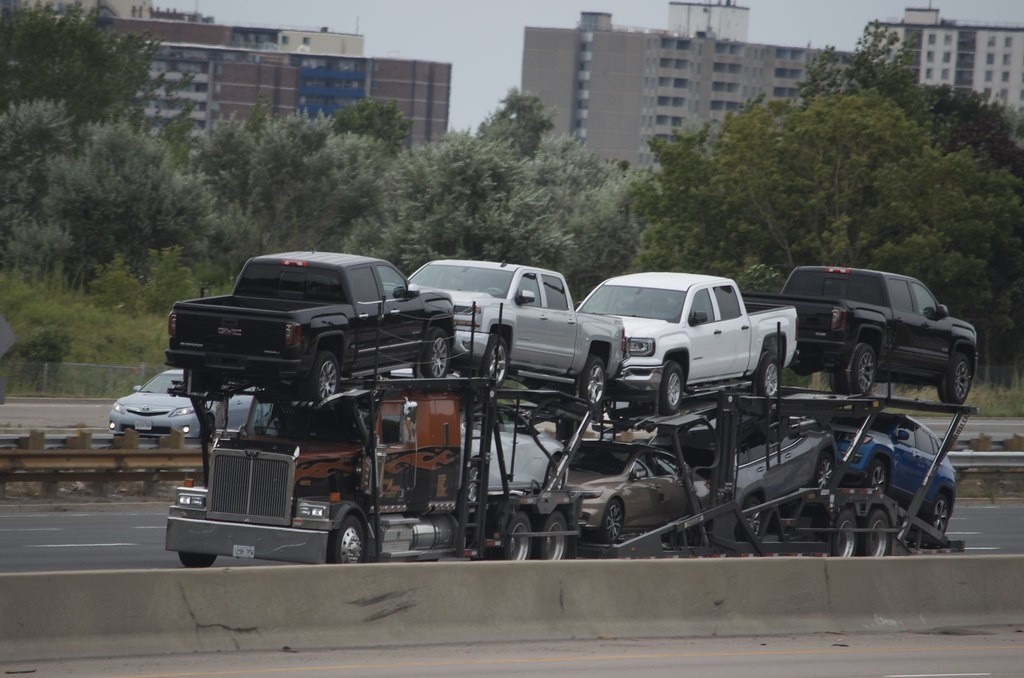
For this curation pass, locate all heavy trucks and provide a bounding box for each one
[159,300,969,557]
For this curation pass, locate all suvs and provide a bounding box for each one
[828,410,956,536]
[652,423,837,542]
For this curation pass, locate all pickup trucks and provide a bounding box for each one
[165,251,456,399]
[741,264,994,408]
[574,272,799,418]
[402,259,626,411]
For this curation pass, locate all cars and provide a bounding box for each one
[521,440,715,544]
[108,369,275,444]
